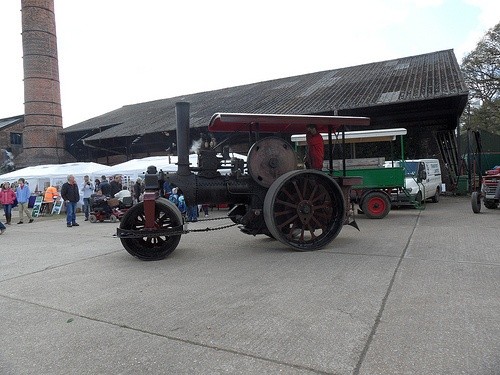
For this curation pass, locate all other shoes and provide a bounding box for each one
[67,224,72,227]
[72,223,79,226]
[17,221,23,224]
[29,219,34,223]
[0,229,6,235]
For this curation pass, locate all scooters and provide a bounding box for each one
[88,194,126,223]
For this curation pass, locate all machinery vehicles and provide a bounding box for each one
[105,99,373,263]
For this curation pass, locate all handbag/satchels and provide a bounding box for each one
[11,198,18,208]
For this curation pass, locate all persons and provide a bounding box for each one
[44,186,58,214]
[61,175,80,226]
[81,175,94,221]
[0,222,6,235]
[93,175,131,214]
[0,182,15,225]
[134,177,145,202]
[163,179,208,222]
[305,124,323,170]
[16,178,34,224]
[0,180,29,208]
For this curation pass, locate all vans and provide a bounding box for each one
[383,158,442,208]
